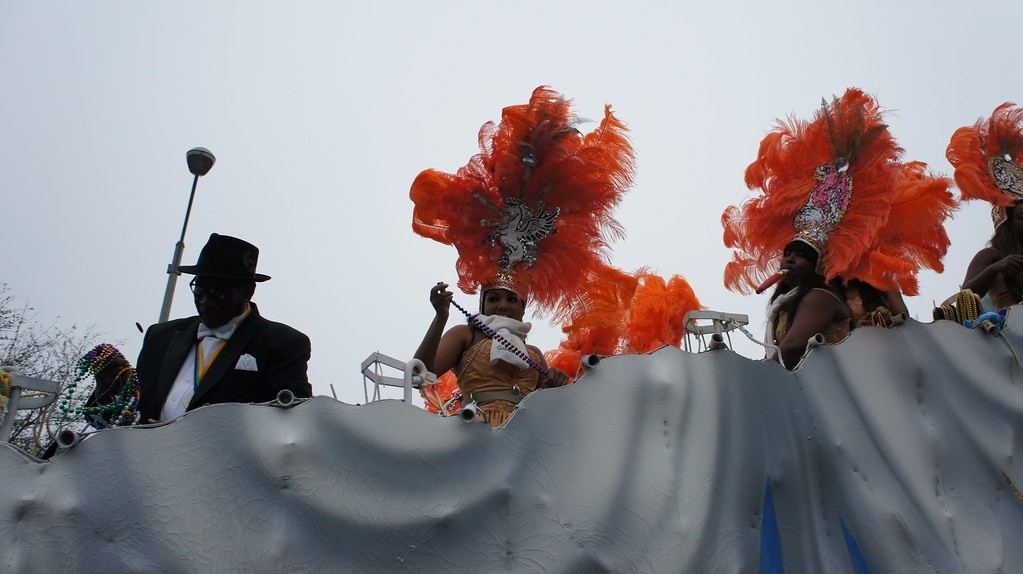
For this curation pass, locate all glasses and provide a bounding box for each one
[190,276,249,302]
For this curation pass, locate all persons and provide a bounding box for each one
[85,233,311,427]
[961,200,1023,311]
[764,237,851,371]
[413,282,569,427]
[833,277,909,328]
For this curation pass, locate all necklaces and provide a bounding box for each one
[60,344,140,428]
[956,291,977,324]
[871,312,886,327]
[940,305,956,323]
[449,299,548,377]
[850,296,852,297]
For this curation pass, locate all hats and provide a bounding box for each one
[178,233,271,282]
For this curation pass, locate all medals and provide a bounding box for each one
[184,396,192,408]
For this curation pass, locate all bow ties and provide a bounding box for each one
[197,322,236,341]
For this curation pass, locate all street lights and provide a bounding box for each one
[157,145,217,326]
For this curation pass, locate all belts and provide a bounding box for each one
[462,391,525,405]
